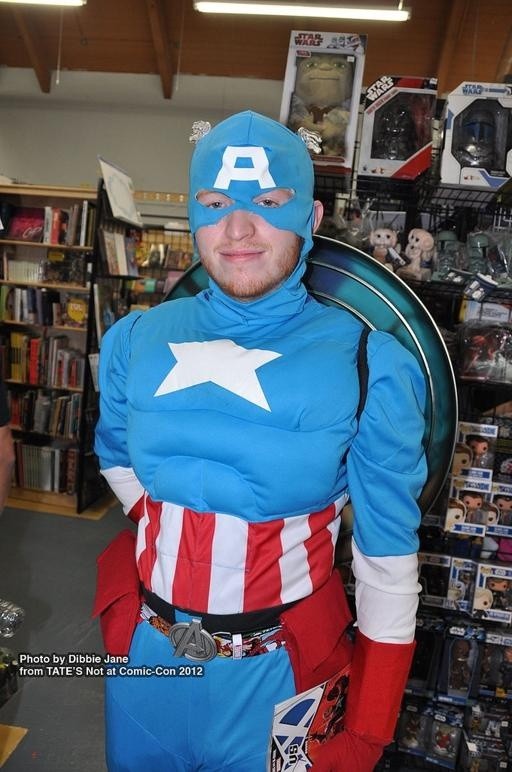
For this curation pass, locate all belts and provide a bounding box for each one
[140,584,309,660]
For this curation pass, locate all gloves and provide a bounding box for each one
[308,628,417,772]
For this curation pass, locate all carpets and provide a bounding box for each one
[0,495,120,521]
[0,723,31,769]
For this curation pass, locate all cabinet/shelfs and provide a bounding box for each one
[313,168,510,771]
[1,176,144,516]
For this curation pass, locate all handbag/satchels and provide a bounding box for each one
[279,568,353,694]
[91,531,138,657]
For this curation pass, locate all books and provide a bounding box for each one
[0,200,139,497]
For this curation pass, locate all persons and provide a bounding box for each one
[92,111,427,772]
[287,54,512,772]
